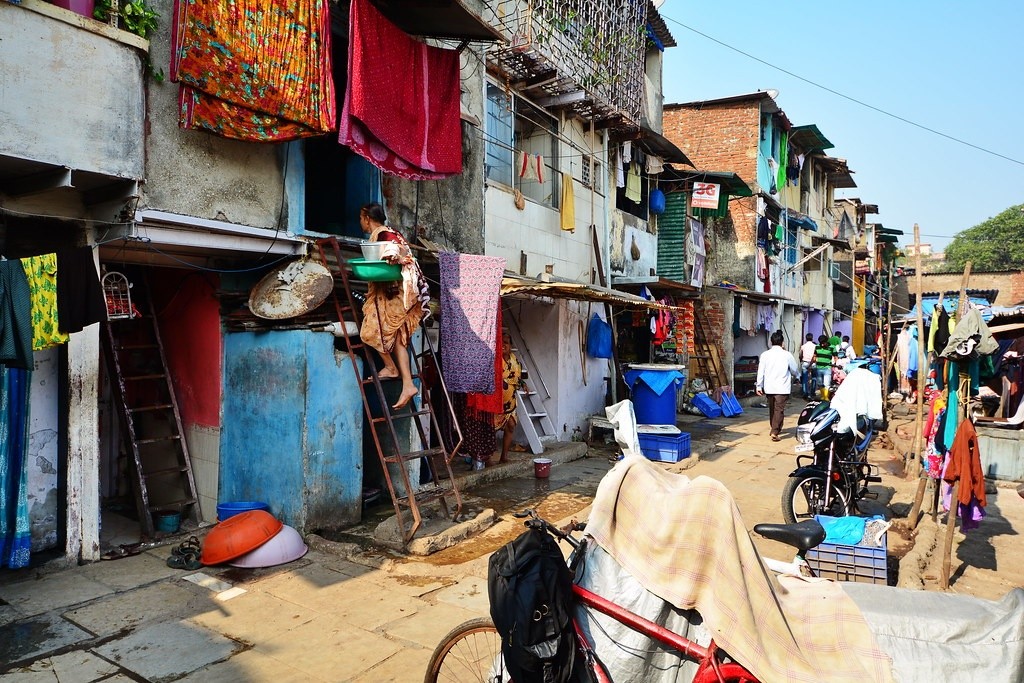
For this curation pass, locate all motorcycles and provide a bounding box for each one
[780,378,890,524]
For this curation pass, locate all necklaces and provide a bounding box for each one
[502,355,511,371]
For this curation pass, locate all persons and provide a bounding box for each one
[756,328,856,440]
[486,333,529,466]
[359,201,430,412]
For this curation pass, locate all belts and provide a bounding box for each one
[803,361,816,363]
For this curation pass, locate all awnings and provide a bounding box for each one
[785,208,818,232]
[637,127,753,212]
[340,0,509,44]
[500,269,686,311]
[863,203,904,243]
[816,154,858,188]
[825,238,853,251]
[790,124,835,157]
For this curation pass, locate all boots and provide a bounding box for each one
[822,388,829,401]
[820,387,825,400]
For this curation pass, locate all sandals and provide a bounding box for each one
[171,536,202,560]
[167,554,202,571]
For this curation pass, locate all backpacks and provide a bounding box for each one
[839,343,851,359]
[488,518,582,683]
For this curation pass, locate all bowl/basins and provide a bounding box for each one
[214,501,271,522]
[346,258,402,282]
[199,510,283,565]
[230,525,308,568]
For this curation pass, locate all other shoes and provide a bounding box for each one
[803,392,808,400]
[770,434,780,440]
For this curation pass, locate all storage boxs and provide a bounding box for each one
[637,433,692,463]
[691,393,721,418]
[719,391,743,417]
[806,514,888,585]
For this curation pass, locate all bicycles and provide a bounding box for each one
[423,506,827,683]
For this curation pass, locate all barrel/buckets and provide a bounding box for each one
[533,458,552,478]
[359,239,395,260]
[157,510,180,533]
